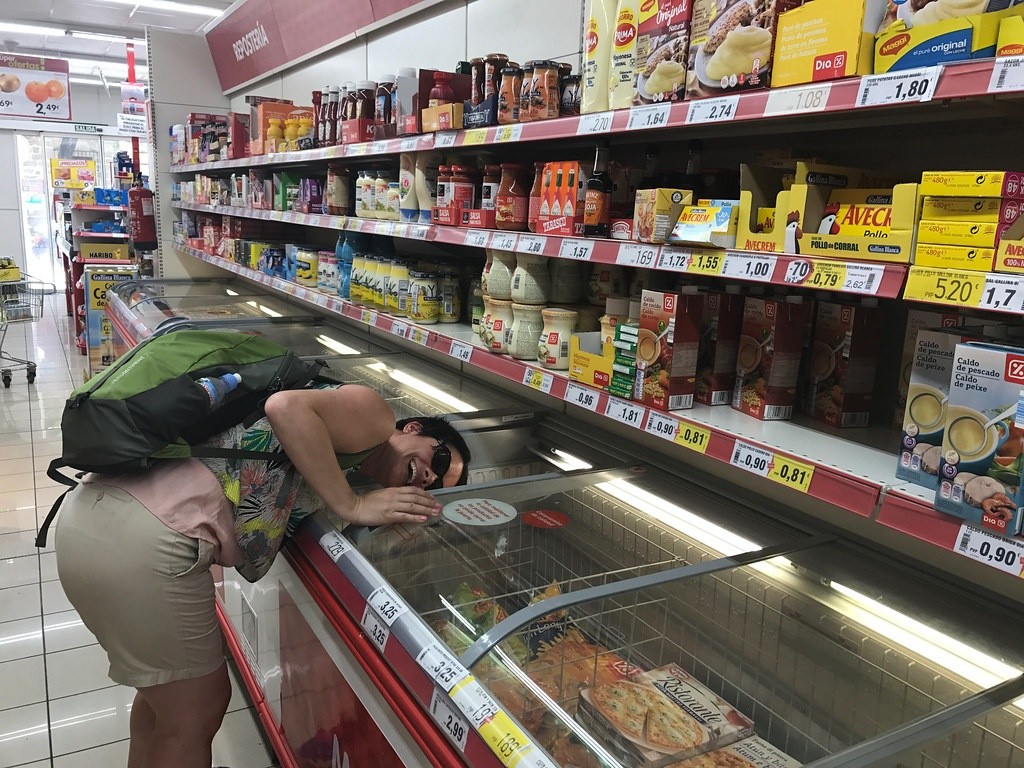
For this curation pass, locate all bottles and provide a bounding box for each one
[680,141,701,206]
[429,72,454,108]
[540,163,552,215]
[198,120,232,162]
[267,117,312,143]
[312,81,347,147]
[550,162,562,215]
[192,373,242,408]
[399,151,439,224]
[562,162,575,216]
[645,142,663,189]
[584,139,611,239]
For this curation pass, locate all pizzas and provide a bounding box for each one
[590,681,703,751]
[661,747,758,768]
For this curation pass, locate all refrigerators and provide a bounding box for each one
[103,277,1024,768]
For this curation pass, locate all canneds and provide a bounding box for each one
[295,53,662,371]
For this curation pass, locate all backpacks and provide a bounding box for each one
[62,330,387,474]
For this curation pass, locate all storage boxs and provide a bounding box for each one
[537,2,1024,535]
[168,33,494,280]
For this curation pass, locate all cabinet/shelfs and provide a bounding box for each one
[56,56,1024,578]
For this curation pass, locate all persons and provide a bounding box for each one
[54,381,471,768]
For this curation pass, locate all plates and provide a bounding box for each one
[694,0,769,88]
[636,36,689,100]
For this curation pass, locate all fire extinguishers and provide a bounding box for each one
[128,172,158,251]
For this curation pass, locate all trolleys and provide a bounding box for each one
[0,271,57,389]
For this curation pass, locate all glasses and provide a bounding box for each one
[424,438,451,491]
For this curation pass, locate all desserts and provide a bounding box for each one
[641,0,777,96]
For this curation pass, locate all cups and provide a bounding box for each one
[808,341,836,385]
[900,383,949,455]
[735,334,761,375]
[937,405,1010,486]
[637,328,661,368]
